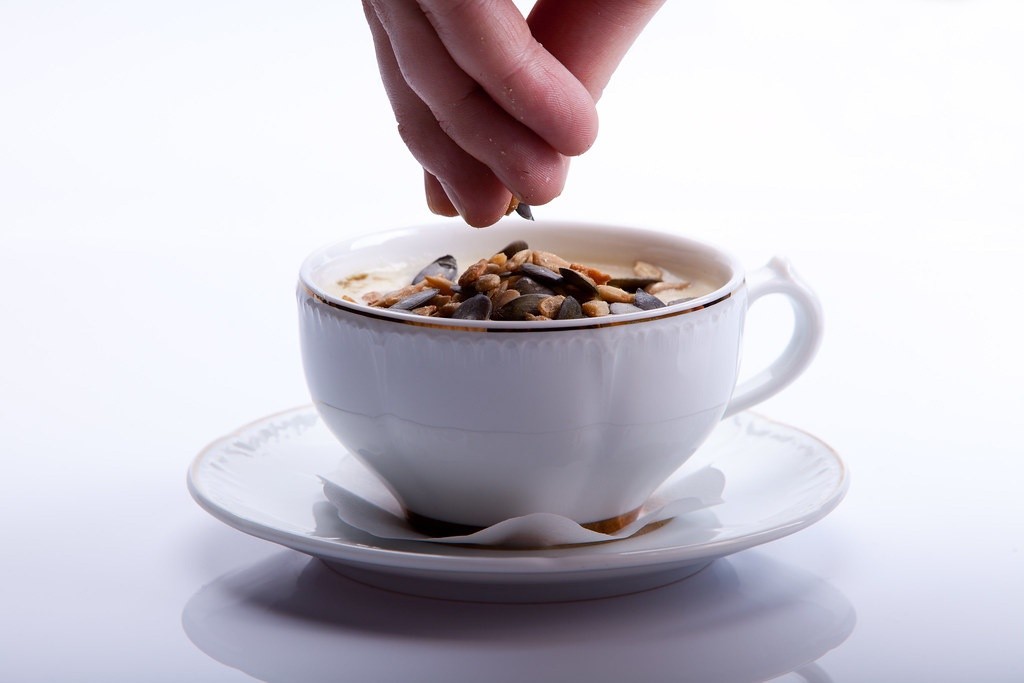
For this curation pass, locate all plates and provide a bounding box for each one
[186,404,849,604]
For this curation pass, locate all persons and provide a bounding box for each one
[361,0,668,228]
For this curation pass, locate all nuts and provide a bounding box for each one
[341,193,693,321]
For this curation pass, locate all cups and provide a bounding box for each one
[295,209,823,537]
